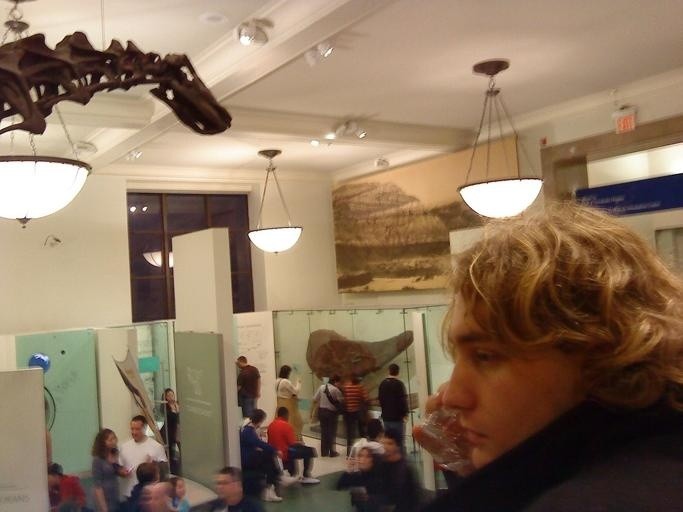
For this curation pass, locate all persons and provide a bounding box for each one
[214,467,266,512]
[46,415,190,512]
[235,356,416,511]
[160,389,179,457]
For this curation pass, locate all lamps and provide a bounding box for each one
[329,119,371,139]
[454,60,544,219]
[0,0,88,228]
[304,42,335,65]
[245,150,301,253]
[141,249,173,269]
[232,18,274,47]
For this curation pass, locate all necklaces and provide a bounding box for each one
[411,203,683,511]
